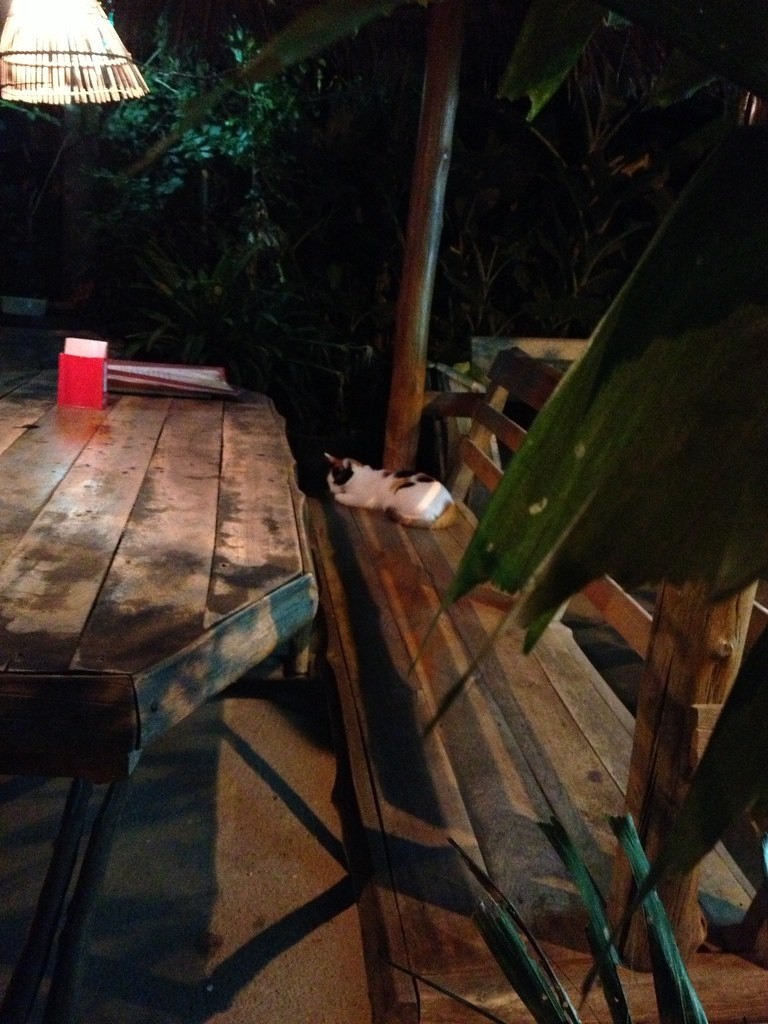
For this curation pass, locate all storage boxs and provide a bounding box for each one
[56,339,109,410]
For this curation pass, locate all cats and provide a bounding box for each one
[321,452,459,530]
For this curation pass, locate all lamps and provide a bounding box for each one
[0,0,150,104]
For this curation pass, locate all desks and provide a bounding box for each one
[0,353,319,1024]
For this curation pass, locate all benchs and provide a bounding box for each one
[288,348,768,1024]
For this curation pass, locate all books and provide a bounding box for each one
[108,359,241,399]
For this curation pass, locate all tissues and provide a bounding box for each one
[57,338,108,410]
